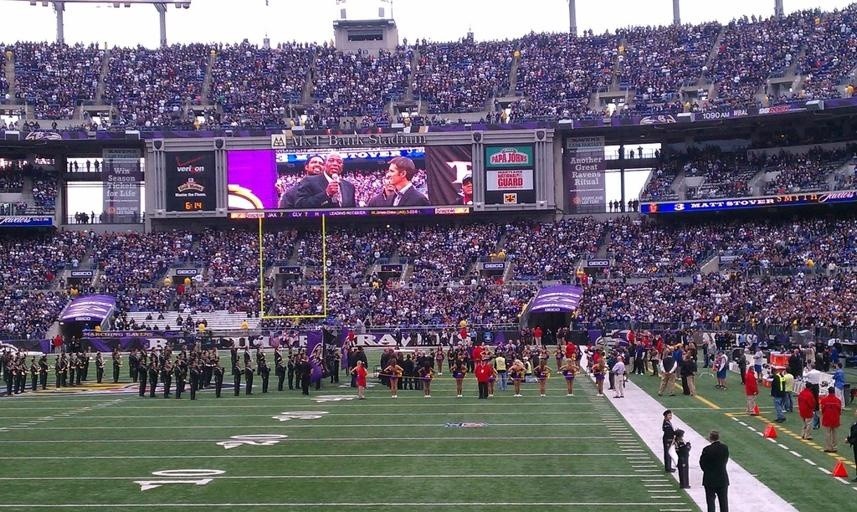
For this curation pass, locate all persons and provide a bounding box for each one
[744,366,758,415]
[769,370,787,424]
[698,430,729,511]
[810,385,820,431]
[661,409,675,473]
[672,427,691,489]
[819,385,841,452]
[1,5,856,411]
[844,422,856,482]
[797,383,815,441]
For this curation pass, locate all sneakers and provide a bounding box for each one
[130,374,339,400]
[748,412,759,416]
[7,376,119,396]
[851,477,857,482]
[631,370,696,396]
[824,449,838,453]
[802,437,814,441]
[774,417,787,423]
[346,370,627,398]
[666,468,691,488]
[715,385,728,389]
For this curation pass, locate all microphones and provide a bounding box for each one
[332,173,339,201]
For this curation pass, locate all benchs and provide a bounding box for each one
[115,310,265,331]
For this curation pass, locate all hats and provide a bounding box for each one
[663,409,673,418]
[674,429,685,436]
